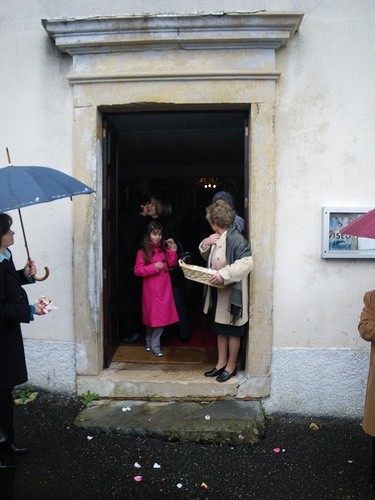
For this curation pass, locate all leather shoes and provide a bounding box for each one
[204,365,227,377]
[0,442,29,456]
[0,461,16,471]
[216,366,237,382]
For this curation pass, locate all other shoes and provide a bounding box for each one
[123,334,145,343]
[180,334,192,342]
[145,347,152,351]
[153,351,163,357]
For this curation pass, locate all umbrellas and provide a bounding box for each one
[0,147,95,281]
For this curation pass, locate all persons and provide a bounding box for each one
[358,291,375,485]
[0,213,47,470]
[119,189,253,383]
